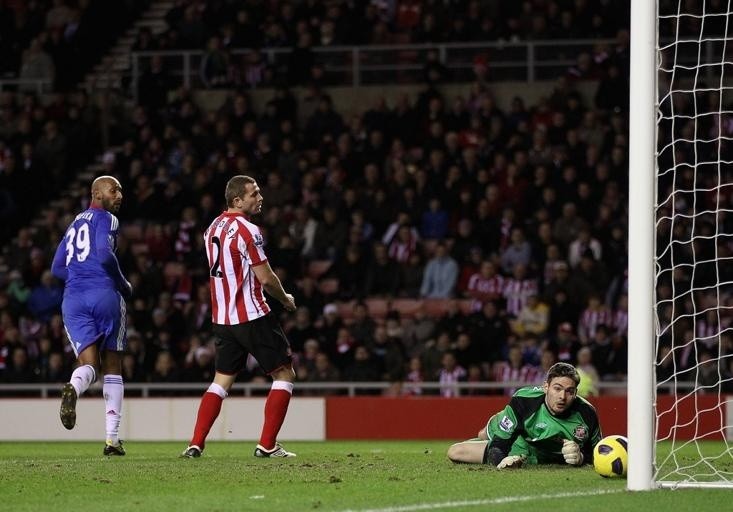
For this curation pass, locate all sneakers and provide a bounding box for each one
[254,443,296,458]
[103,439,125,455]
[58,382,76,430]
[182,445,202,457]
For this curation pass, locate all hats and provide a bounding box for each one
[322,303,338,315]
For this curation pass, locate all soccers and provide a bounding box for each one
[593,435,627,478]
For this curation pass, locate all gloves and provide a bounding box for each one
[494,453,526,470]
[561,438,583,465]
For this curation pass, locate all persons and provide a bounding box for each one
[49,175,136,457]
[179,176,300,458]
[447,360,602,470]
